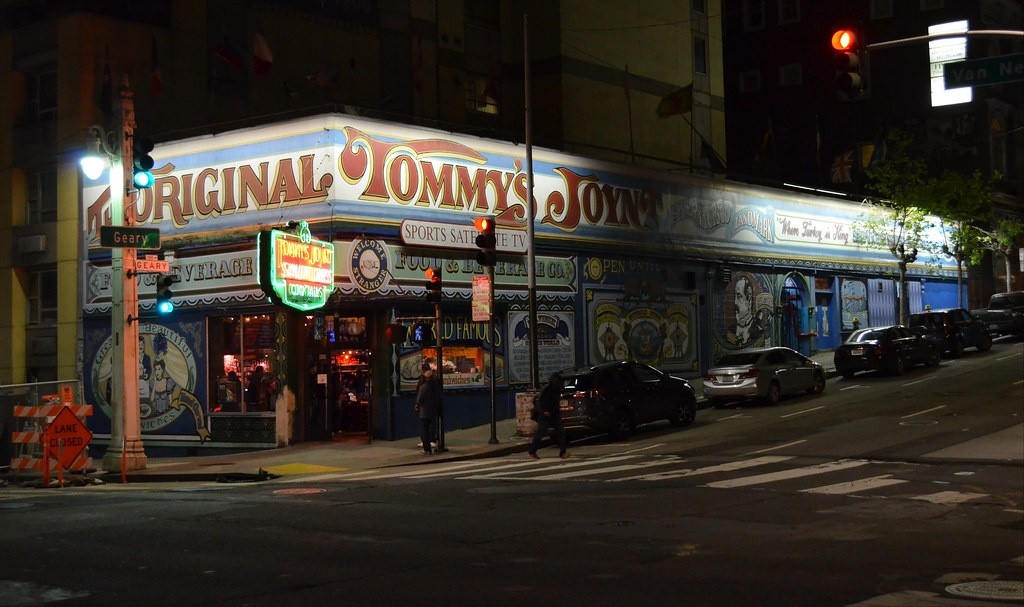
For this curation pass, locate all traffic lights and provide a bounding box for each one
[474,214,497,267]
[132,137,155,188]
[156,275,173,315]
[830,19,873,106]
[385,324,402,344]
[425,266,442,305]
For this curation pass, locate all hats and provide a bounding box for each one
[423,370,434,377]
[421,363,430,370]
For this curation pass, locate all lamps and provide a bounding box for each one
[305,310,316,319]
[223,336,235,365]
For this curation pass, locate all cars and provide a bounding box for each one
[834,324,942,379]
[702,346,826,408]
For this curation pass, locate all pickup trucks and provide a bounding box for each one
[969,290,1024,340]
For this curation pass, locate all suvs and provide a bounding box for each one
[529,359,697,443]
[908,307,992,359]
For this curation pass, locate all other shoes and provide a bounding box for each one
[429,442,436,447]
[418,442,424,447]
[560,452,571,458]
[528,451,539,459]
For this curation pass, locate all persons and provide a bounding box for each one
[528,373,571,459]
[246,366,276,412]
[222,370,241,413]
[331,365,365,435]
[415,363,441,455]
